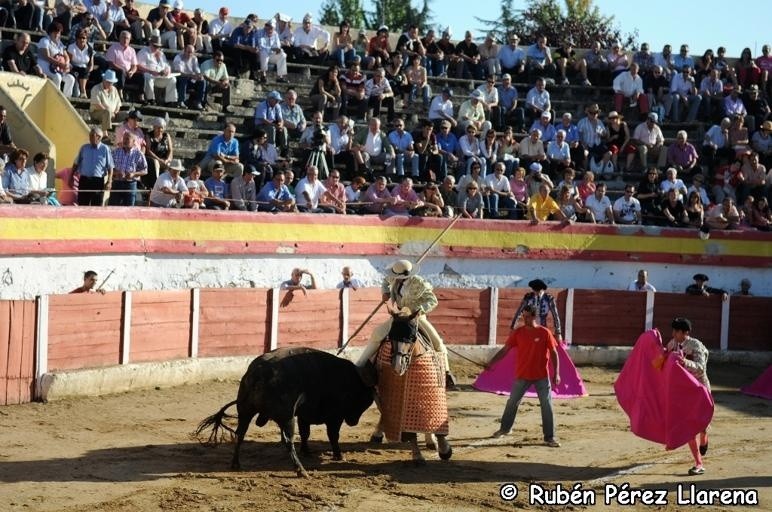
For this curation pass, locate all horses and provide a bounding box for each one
[372,303,453,468]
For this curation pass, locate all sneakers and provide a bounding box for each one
[688,466,706,474]
[699,432,709,455]
[492,428,513,438]
[444,375,457,388]
[543,439,562,448]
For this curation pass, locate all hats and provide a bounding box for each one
[384,259,420,280]
[128,110,143,122]
[584,103,659,125]
[692,274,709,282]
[442,73,512,103]
[149,35,163,48]
[359,25,521,42]
[731,84,772,133]
[101,69,118,83]
[527,279,547,292]
[671,316,692,332]
[153,0,313,29]
[267,91,282,102]
[167,158,262,177]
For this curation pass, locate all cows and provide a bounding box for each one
[184,344,378,479]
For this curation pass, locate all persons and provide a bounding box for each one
[335,266,369,290]
[733,278,754,295]
[481,303,564,450]
[684,273,729,302]
[65,270,106,298]
[662,316,714,476]
[279,266,316,298]
[510,276,564,345]
[626,268,656,293]
[1,0,772,239]
[356,258,459,388]
